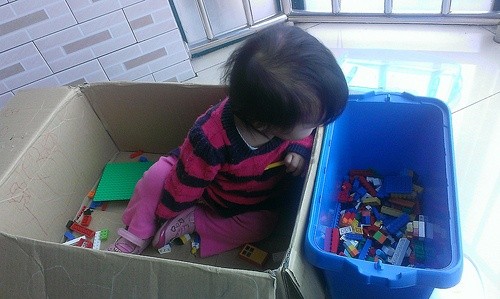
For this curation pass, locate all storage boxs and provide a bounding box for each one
[1,81,325,299]
[304,90,465,299]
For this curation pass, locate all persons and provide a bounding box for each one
[106,25,349,255]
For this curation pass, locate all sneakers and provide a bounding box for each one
[150,205,195,249]
[109,228,151,254]
